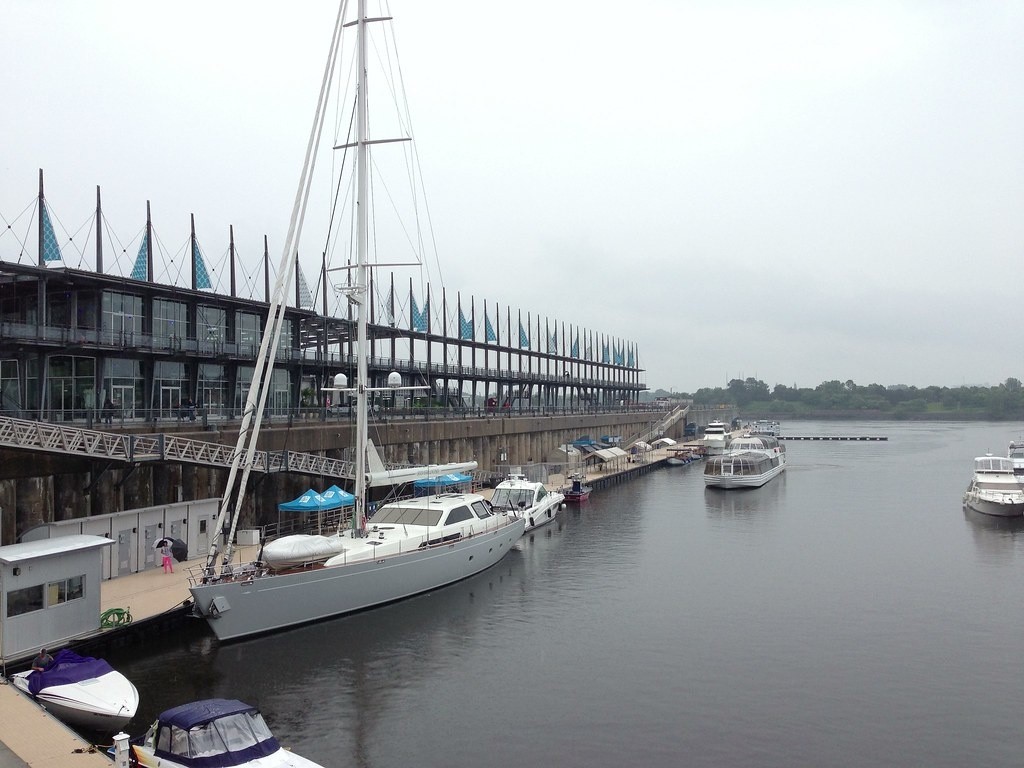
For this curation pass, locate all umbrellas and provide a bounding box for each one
[171,539,187,563]
[152,537,175,549]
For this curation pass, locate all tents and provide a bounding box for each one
[414,473,473,498]
[278,485,354,535]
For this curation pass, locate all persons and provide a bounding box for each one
[161,542,173,573]
[327,399,334,418]
[32,648,53,671]
[188,399,197,422]
[102,399,114,428]
[503,401,509,416]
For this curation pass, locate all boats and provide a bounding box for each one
[12,648,140,735]
[188,0,525,642]
[488,473,566,533]
[962,436,1024,517]
[667,444,705,465]
[108,696,325,768]
[703,435,786,488]
[564,473,593,502]
